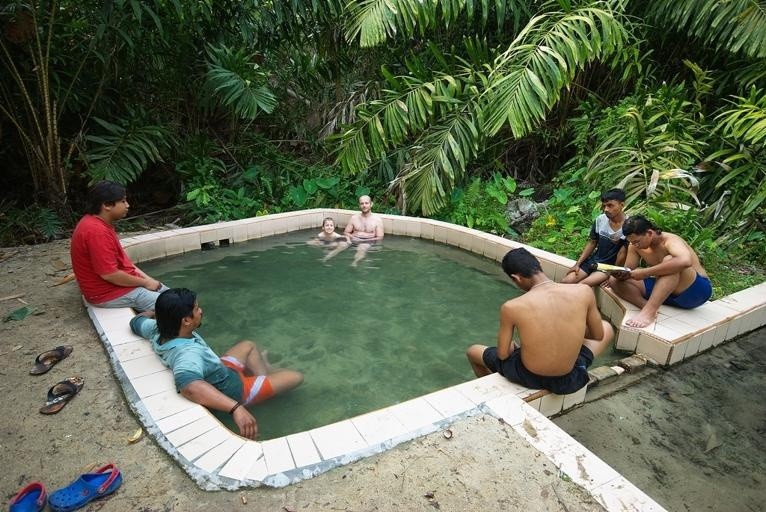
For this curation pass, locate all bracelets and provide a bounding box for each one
[229,402,241,415]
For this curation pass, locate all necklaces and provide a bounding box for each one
[530,279,553,291]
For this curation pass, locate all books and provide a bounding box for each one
[596,262,631,274]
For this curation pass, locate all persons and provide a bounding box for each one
[71,180,171,314]
[321,195,384,268]
[608,216,712,328]
[558,188,631,287]
[307,217,352,250]
[466,248,615,395]
[130,287,304,442]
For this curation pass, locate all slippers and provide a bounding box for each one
[9,479,49,512]
[47,461,123,512]
[28,344,75,376]
[38,375,85,415]
